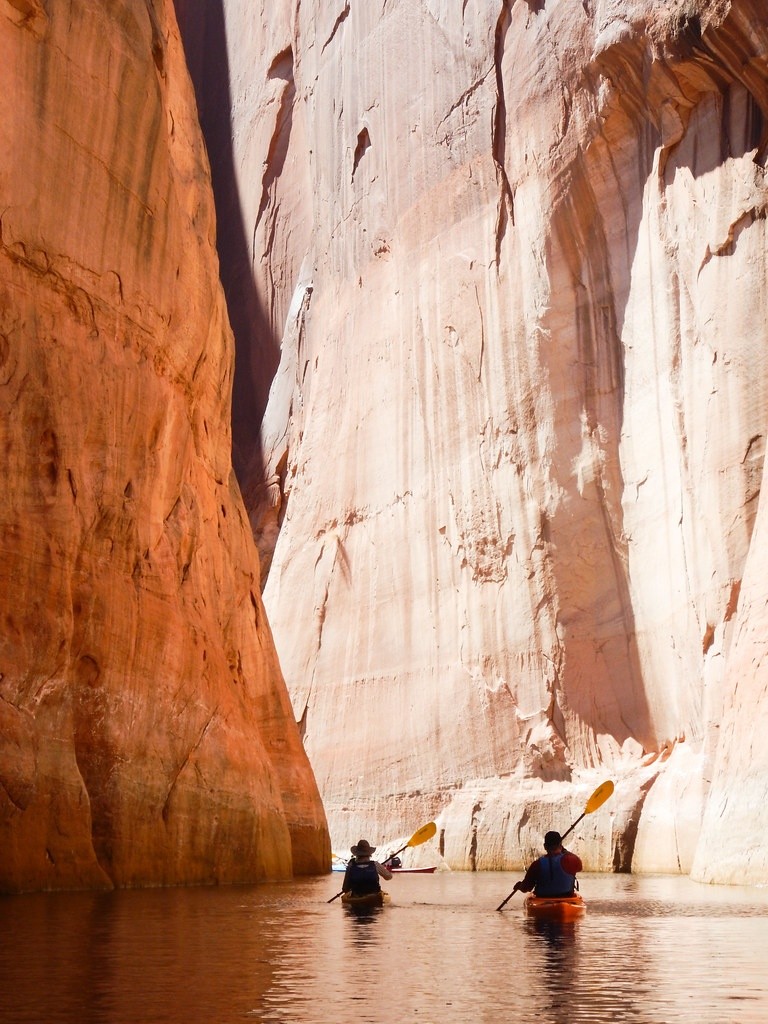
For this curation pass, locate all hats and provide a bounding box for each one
[350,839,376,857]
[387,850,395,855]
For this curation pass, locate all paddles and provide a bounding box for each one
[331,854,346,860]
[326,821,438,904]
[496,779,615,912]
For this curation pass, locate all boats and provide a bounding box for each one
[341,891,390,908]
[523,891,586,925]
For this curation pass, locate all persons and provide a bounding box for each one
[344,855,354,867]
[513,831,582,897]
[383,850,402,869]
[342,840,392,897]
